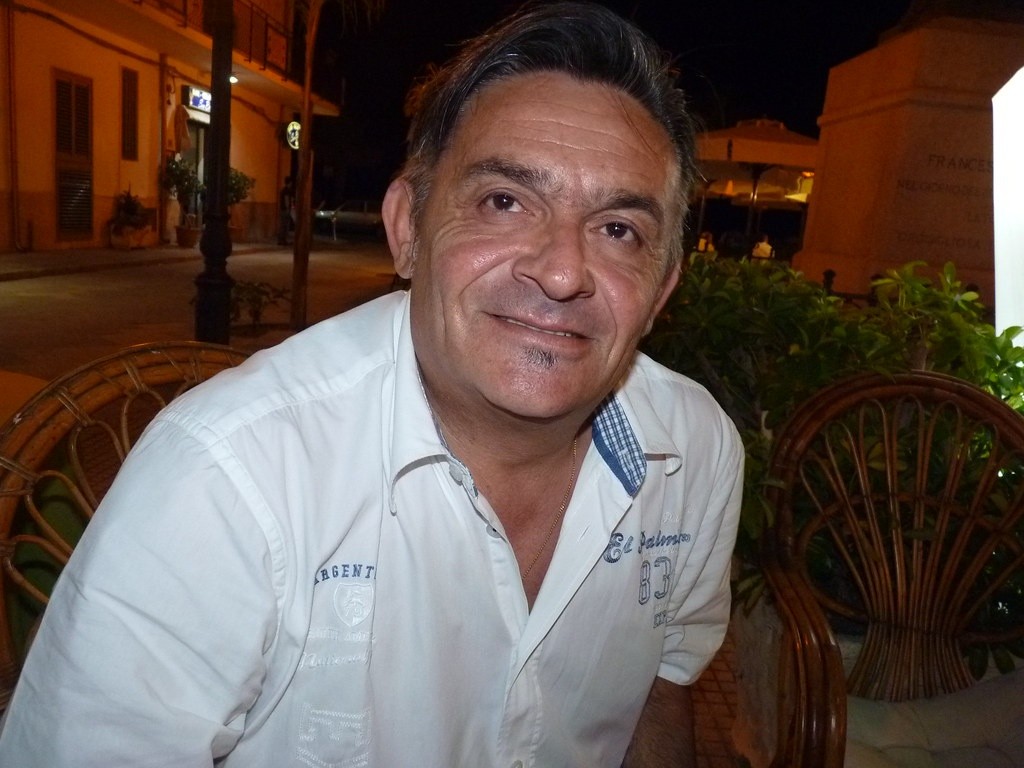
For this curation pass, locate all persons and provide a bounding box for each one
[0,0,746,768]
[751,233,775,263]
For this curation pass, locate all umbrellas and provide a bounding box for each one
[691,119,820,243]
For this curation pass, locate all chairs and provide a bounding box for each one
[688,190,809,267]
[0,346,1024,768]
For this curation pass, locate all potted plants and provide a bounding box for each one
[164,157,205,248]
[228,167,256,242]
[108,189,151,248]
